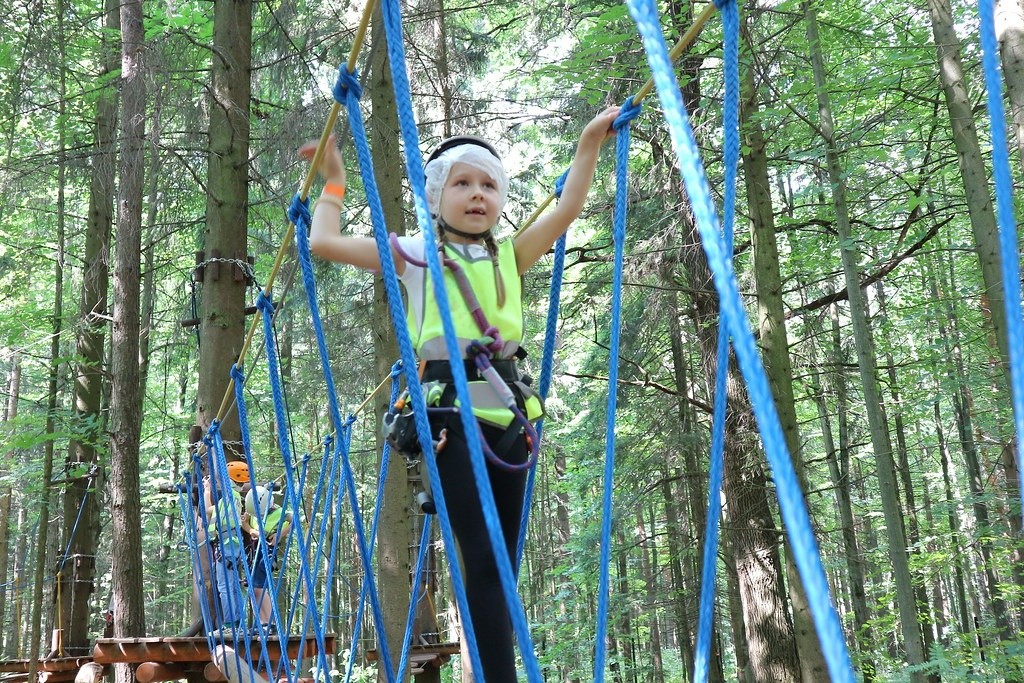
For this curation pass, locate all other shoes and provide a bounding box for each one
[208,624,226,636]
[216,627,244,635]
[247,623,278,635]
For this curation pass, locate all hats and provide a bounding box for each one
[424,144,509,217]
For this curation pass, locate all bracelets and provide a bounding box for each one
[312,196,344,213]
[322,181,345,199]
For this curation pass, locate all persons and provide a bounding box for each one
[200,461,251,638]
[240,486,294,637]
[298,104,623,683]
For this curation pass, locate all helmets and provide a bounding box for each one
[423,135,502,185]
[226,461,250,483]
[245,486,274,517]
